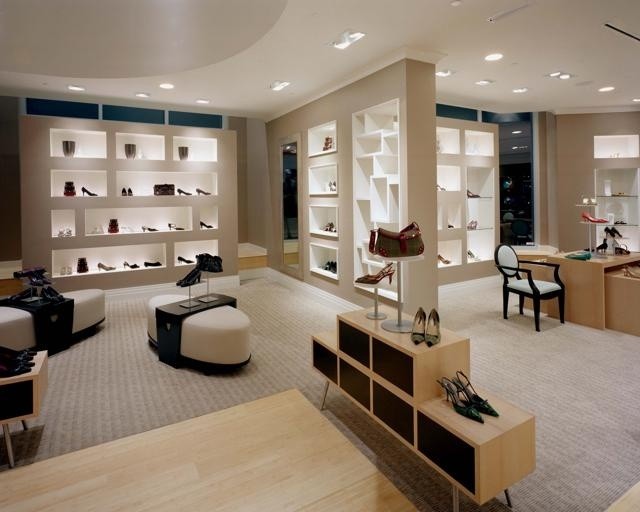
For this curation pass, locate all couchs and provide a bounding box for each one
[147,293,253,375]
[0,288,106,352]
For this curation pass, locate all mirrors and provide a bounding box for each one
[277,133,307,280]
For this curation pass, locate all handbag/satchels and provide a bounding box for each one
[195,251,224,274]
[368,221,423,258]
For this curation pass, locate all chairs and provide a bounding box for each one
[494,244,565,331]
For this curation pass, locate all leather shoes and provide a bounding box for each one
[8,264,65,304]
[0,347,38,380]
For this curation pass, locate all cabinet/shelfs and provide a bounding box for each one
[0,350,48,467]
[311,304,535,512]
[307,96,402,308]
[19,113,240,294]
[435,114,500,287]
[592,134,640,253]
[546,246,640,337]
[509,245,559,314]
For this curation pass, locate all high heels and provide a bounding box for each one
[467,188,481,200]
[437,254,450,265]
[466,219,478,230]
[59,188,215,289]
[411,306,440,347]
[318,132,339,276]
[623,265,640,279]
[578,194,631,255]
[436,370,499,424]
[355,260,394,285]
[436,185,447,192]
[467,249,481,262]
